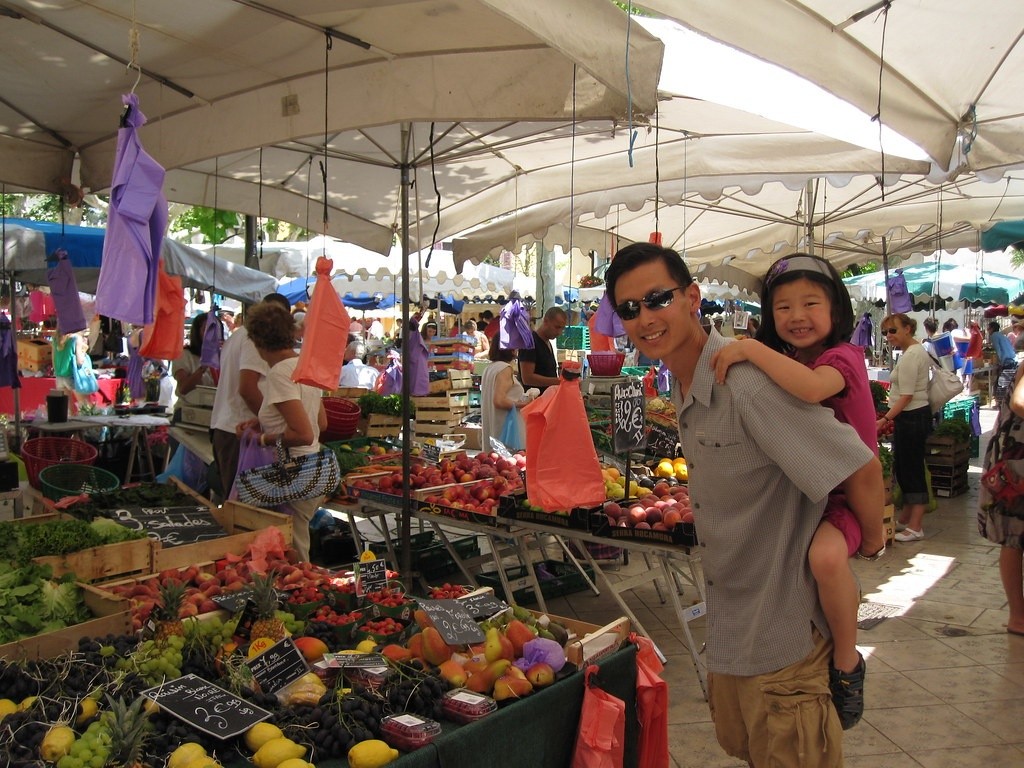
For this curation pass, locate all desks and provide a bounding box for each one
[0,363,129,418]
[8,414,170,489]
[321,497,710,702]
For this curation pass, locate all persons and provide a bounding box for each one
[0,284,23,317]
[229,306,327,565]
[922,317,1024,407]
[335,316,537,388]
[170,314,225,456]
[876,313,964,542]
[483,333,538,455]
[746,318,759,336]
[608,242,885,768]
[976,358,1024,636]
[206,294,292,496]
[518,307,567,388]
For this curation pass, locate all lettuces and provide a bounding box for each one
[0,513,147,645]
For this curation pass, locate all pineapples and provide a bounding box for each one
[152,576,187,645]
[240,568,285,643]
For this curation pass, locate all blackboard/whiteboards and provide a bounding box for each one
[455,591,510,618]
[246,636,313,700]
[139,672,274,739]
[355,559,390,597]
[414,596,486,645]
[107,504,229,548]
[210,582,262,612]
[322,651,389,668]
[611,382,647,456]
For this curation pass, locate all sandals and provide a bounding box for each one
[829,649,867,729]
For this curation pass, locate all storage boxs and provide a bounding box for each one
[924,396,975,499]
[17,338,52,373]
[326,331,701,606]
[0,489,294,664]
[883,461,895,545]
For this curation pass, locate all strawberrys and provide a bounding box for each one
[278,562,467,636]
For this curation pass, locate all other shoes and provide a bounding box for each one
[895,528,924,541]
[892,521,908,532]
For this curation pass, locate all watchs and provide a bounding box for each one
[857,546,885,562]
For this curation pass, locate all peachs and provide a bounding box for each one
[105,564,255,627]
[340,443,526,515]
[600,481,694,533]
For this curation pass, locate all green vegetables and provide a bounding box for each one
[867,380,889,410]
[934,417,970,444]
[879,445,894,479]
[356,389,416,427]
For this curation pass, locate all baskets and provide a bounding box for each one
[232,431,342,507]
[319,395,362,439]
[20,438,99,488]
[587,352,626,375]
[37,462,121,502]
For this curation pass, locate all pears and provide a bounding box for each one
[602,457,688,498]
[215,644,236,671]
[293,602,568,701]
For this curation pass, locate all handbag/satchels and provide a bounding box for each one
[155,444,204,493]
[52,334,99,395]
[918,348,964,414]
[981,460,1024,511]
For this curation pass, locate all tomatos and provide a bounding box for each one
[875,412,894,437]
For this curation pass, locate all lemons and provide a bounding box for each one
[17,696,34,712]
[0,698,16,724]
[348,740,399,768]
[243,722,285,752]
[252,738,307,767]
[75,698,97,724]
[39,726,76,761]
[185,756,225,768]
[168,743,206,768]
[276,758,315,768]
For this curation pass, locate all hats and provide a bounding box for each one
[217,305,234,317]
[713,317,723,322]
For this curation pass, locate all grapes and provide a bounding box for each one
[0,609,452,768]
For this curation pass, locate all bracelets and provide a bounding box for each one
[883,416,889,421]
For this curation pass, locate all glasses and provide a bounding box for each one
[881,325,905,337]
[613,284,686,321]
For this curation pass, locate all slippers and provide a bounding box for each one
[1006,627,1024,637]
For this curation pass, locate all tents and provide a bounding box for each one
[0,0,1023,312]
